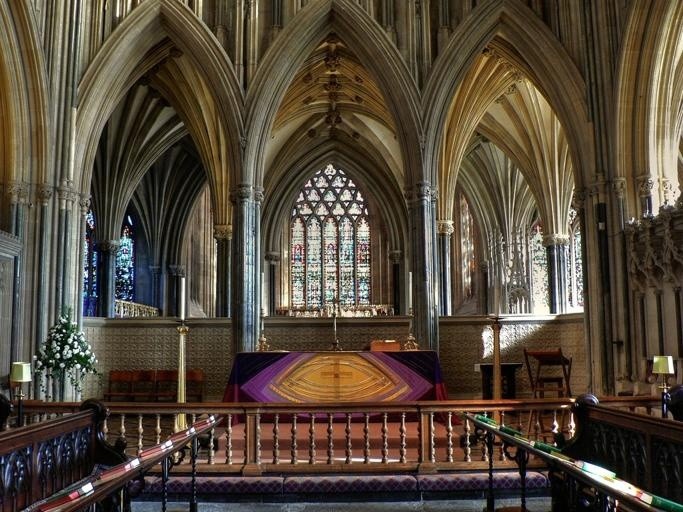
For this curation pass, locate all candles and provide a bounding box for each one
[407,271,414,310]
[262,318,264,331]
[181,277,185,322]
[261,273,264,309]
[334,318,337,330]
[492,285,501,318]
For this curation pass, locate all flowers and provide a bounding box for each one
[32,304,97,375]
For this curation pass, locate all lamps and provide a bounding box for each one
[651,356,676,418]
[9,360,33,425]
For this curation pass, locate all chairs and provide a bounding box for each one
[101,368,206,404]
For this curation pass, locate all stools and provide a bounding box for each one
[537,376,564,399]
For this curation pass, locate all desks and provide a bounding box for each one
[236,352,438,423]
